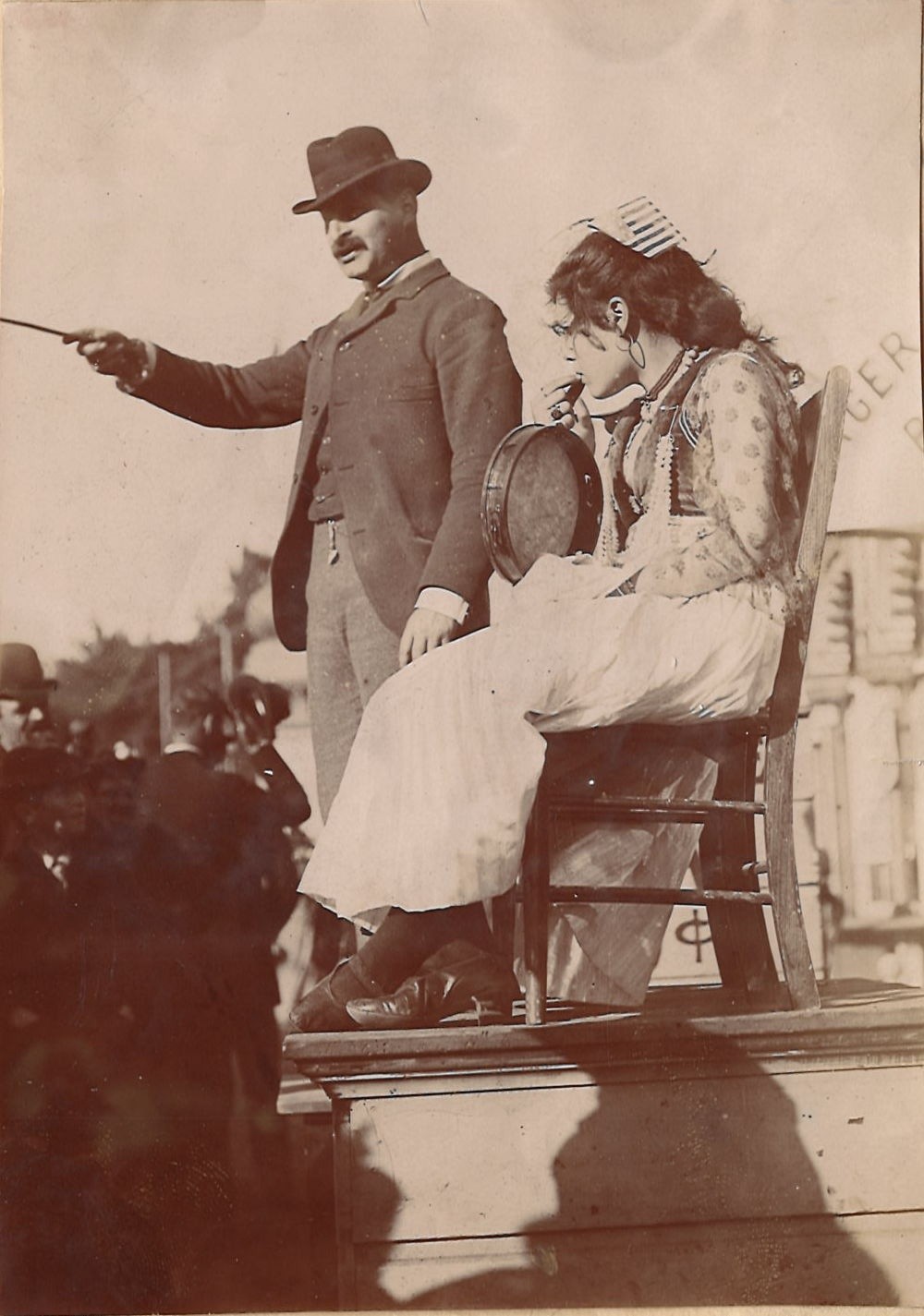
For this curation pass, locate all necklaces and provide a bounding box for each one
[640,345,703,424]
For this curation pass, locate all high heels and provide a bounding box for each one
[288,961,384,1031]
[346,951,521,1026]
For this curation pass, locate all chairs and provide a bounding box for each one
[485,365,854,1024]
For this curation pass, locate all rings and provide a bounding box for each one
[550,408,562,419]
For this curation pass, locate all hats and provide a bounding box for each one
[0,643,60,696]
[229,675,290,739]
[292,126,433,214]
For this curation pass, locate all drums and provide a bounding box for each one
[478,421,605,585]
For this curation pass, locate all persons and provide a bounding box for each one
[290,193,805,1032]
[61,125,525,953]
[0,642,309,1316]
